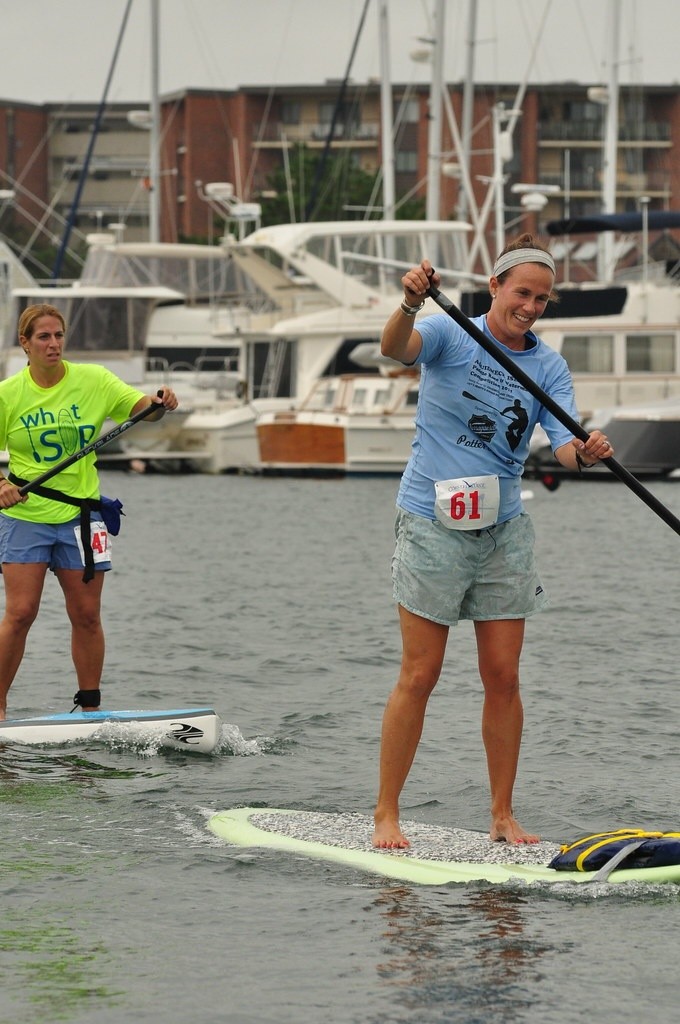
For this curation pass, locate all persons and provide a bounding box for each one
[351,237,613,852]
[0,295,176,721]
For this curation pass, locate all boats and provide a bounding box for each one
[1,706,221,757]
[207,804,679,890]
[0,205,680,481]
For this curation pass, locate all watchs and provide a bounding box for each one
[572,450,597,474]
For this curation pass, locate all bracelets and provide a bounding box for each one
[397,297,427,316]
